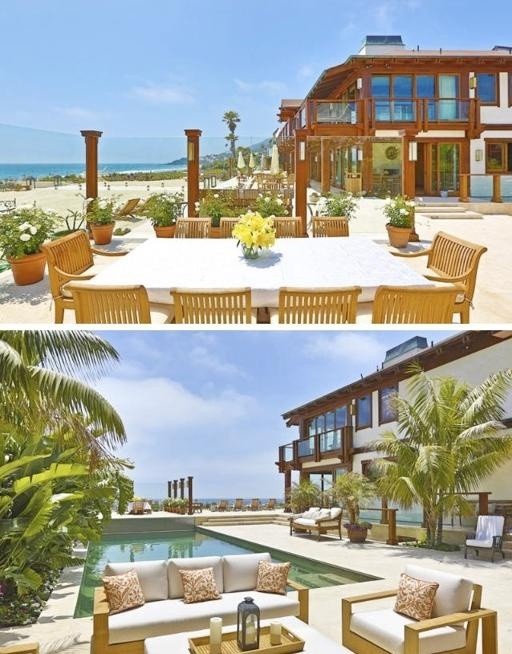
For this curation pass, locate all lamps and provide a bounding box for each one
[407,139,418,163]
[473,146,482,163]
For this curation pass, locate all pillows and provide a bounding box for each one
[99,567,146,616]
[177,566,223,603]
[393,571,440,625]
[254,559,293,596]
[301,506,342,520]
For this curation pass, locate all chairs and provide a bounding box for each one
[60,281,178,325]
[460,511,506,563]
[388,230,489,324]
[269,286,362,324]
[356,282,458,324]
[167,286,273,324]
[116,193,156,219]
[189,497,280,515]
[41,226,131,324]
[170,174,350,239]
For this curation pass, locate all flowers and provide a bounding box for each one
[377,191,426,229]
[0,206,60,262]
[228,205,279,249]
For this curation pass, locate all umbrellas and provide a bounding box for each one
[237,150,246,179]
[271,143,280,175]
[260,153,266,172]
[248,150,255,178]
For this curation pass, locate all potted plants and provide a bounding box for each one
[341,518,374,543]
[84,200,116,245]
[142,199,181,240]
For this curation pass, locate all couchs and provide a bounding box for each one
[86,550,311,654]
[286,506,343,542]
[341,564,500,653]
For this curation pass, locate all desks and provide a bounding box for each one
[87,234,435,324]
[124,500,152,514]
[138,613,355,654]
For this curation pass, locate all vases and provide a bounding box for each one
[4,247,52,286]
[384,220,415,248]
[238,241,263,258]
